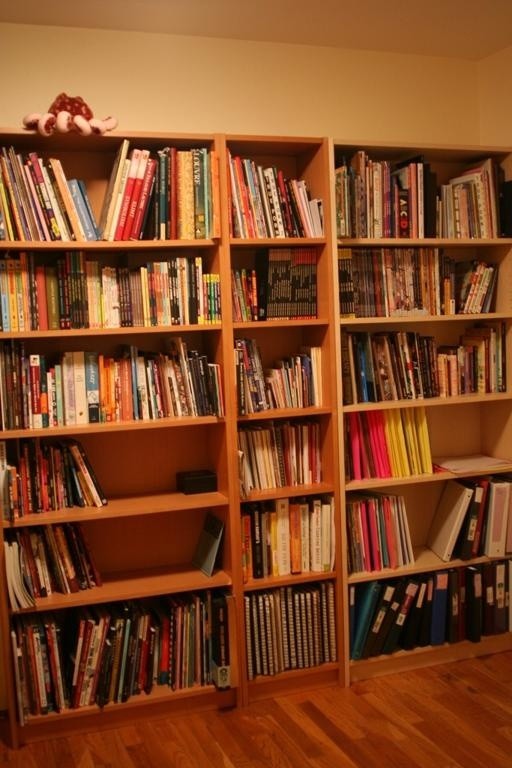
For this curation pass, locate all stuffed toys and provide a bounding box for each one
[22,92,120,135]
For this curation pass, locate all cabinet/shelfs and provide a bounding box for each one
[332,126,511,684]
[221,131,348,711]
[0,126,246,750]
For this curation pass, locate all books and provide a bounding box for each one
[237,422,321,501]
[335,149,511,238]
[233,245,320,322]
[426,454,512,562]
[348,562,510,661]
[338,247,506,406]
[344,407,434,577]
[234,336,324,417]
[242,495,336,585]
[244,578,337,682]
[228,144,328,238]
[2,137,229,726]
